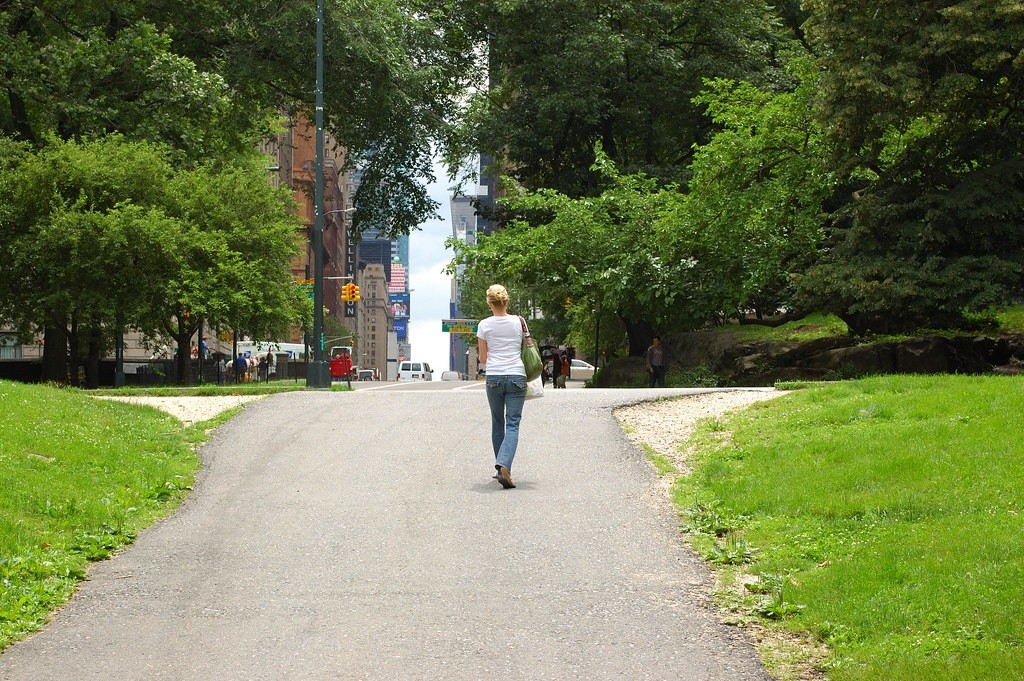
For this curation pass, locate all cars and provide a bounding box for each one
[544,358,601,381]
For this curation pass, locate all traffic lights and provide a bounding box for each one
[341,284,350,302]
[355,286,360,302]
[566,297,573,311]
[349,341,354,350]
[350,284,356,300]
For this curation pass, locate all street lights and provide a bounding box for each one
[306,207,356,387]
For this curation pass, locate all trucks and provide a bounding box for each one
[235,341,312,381]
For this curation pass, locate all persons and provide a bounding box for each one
[646,336,668,388]
[477,285,529,488]
[475,369,480,380]
[551,348,572,389]
[392,305,407,316]
[190,335,248,384]
[258,357,269,382]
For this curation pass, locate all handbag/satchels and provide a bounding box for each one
[523,374,544,400]
[519,315,543,382]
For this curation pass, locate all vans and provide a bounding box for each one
[358,369,376,382]
[442,371,462,381]
[398,361,434,381]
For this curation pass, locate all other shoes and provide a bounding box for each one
[493,468,515,488]
